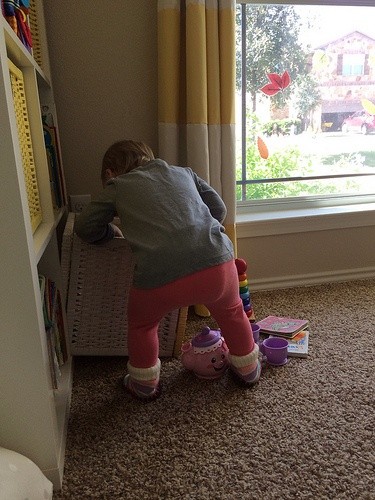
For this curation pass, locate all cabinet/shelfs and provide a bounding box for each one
[0,1,71,490]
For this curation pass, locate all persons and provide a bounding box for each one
[75,138,262,403]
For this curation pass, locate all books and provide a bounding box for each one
[257,312,310,338]
[36,272,69,394]
[268,330,309,358]
[38,102,66,212]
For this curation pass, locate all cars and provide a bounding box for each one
[341,110,375,134]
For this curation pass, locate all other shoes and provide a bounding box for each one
[227,344,263,385]
[124,358,161,400]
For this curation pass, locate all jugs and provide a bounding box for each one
[181,326,229,379]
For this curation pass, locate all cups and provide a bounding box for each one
[251,323,260,343]
[260,337,289,365]
